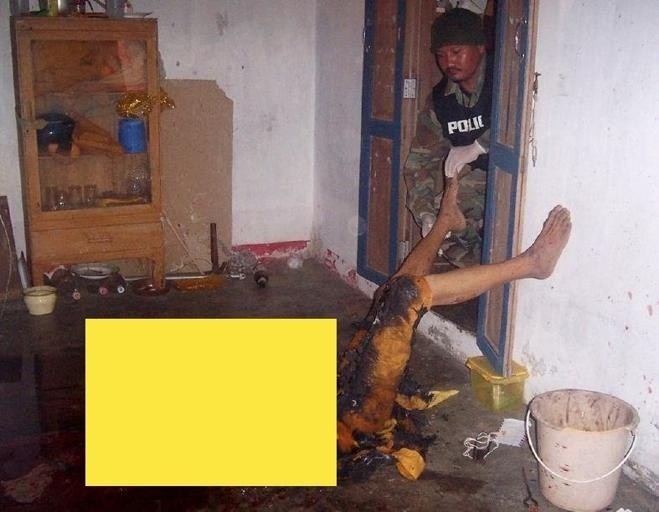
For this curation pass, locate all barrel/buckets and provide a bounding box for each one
[523,386,643,512]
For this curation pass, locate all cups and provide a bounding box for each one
[44,184,97,212]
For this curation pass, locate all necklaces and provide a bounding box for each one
[459,88,474,120]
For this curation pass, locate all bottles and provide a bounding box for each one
[254,261,269,286]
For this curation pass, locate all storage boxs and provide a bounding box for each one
[465,351,529,412]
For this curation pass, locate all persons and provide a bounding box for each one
[338,175,571,454]
[404,7,495,269]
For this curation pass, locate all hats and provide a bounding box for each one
[429,8,484,53]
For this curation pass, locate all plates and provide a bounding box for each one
[70,260,120,279]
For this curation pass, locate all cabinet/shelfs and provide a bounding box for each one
[11,14,166,292]
